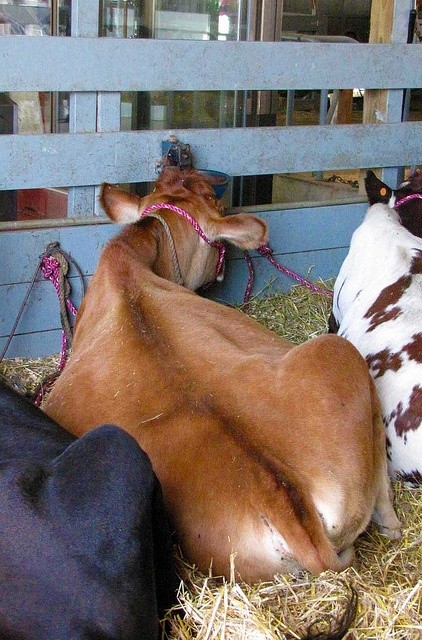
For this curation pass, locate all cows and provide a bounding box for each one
[0,373,180,635]
[37,160,401,586]
[326,167,421,490]
